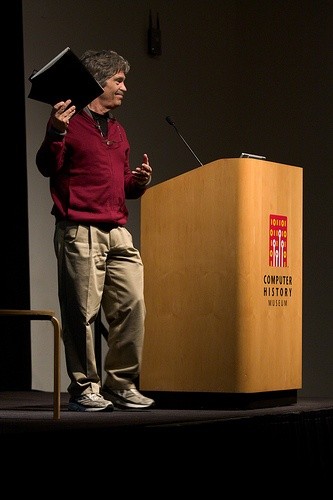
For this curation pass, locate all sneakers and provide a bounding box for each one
[68,393,113,412]
[98,387,154,409]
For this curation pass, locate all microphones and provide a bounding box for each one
[166,117,203,166]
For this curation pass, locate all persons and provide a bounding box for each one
[35,49,154,412]
